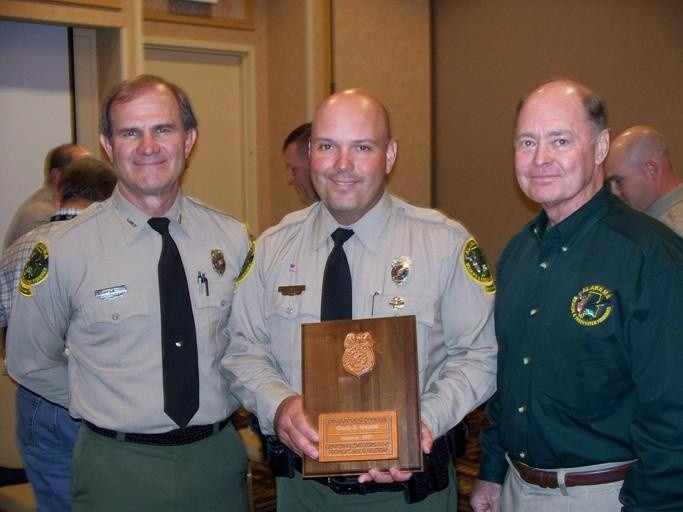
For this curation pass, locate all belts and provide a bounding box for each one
[511,460,632,489]
[83,418,229,445]
[293,454,406,495]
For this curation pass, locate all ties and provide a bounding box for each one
[320,228,355,321]
[148,217,199,428]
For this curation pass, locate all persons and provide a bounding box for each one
[468,78,683,512]
[281,122,321,205]
[4,144,91,252]
[604,125,683,238]
[0,158,117,512]
[220,88,498,512]
[5,75,253,512]
